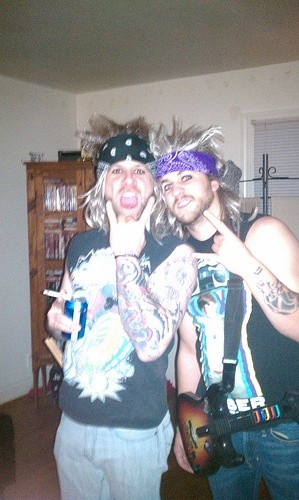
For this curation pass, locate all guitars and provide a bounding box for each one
[174,383,299,478]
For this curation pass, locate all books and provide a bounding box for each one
[45,270,65,313]
[44,218,78,260]
[44,184,78,211]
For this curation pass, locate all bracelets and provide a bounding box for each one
[115,252,139,259]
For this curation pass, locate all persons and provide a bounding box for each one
[159,136,299,500]
[47,135,198,500]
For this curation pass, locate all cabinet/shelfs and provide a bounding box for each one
[25,159,97,408]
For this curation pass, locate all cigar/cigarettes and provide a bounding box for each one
[43,289,71,300]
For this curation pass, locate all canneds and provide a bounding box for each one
[62,296,87,341]
